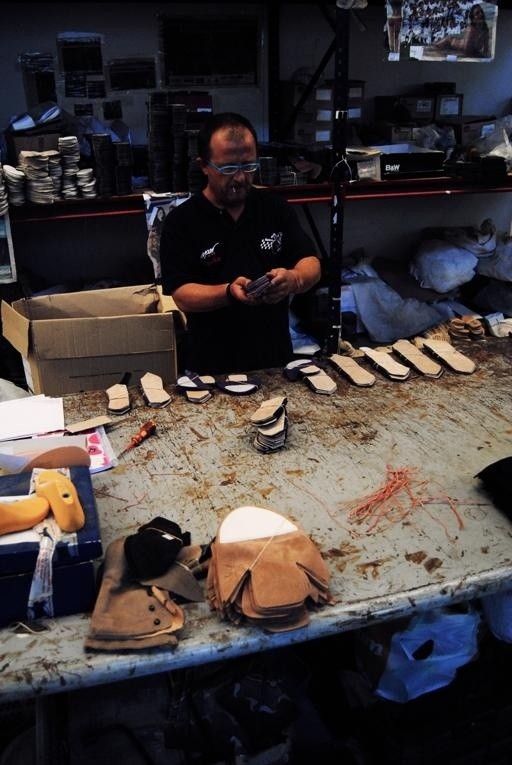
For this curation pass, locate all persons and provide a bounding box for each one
[400,0,492,57]
[157,110,324,379]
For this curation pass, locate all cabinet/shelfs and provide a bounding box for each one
[1,0,512,354]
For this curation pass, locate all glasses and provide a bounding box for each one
[207,161,262,176]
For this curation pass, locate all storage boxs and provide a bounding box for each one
[290,64,497,148]
[0,283,187,397]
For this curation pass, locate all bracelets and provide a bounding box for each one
[224,285,240,306]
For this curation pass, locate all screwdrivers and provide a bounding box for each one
[117,419,157,460]
